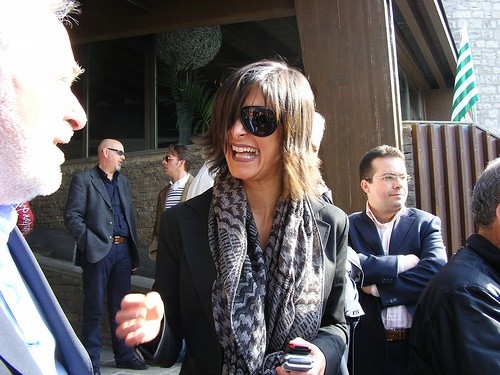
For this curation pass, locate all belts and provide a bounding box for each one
[112,235,130,245]
[386,330,409,341]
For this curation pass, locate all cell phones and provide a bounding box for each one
[285,344,311,355]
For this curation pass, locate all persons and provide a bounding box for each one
[115,60,350,375]
[345,145,448,375]
[147,143,195,260]
[0,0,94,375]
[63,138,148,375]
[407,156,500,375]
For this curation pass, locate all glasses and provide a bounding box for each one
[163,156,183,162]
[227,105,282,137]
[102,147,125,157]
[365,174,412,184]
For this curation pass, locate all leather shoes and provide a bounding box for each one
[93,363,100,375]
[117,356,149,370]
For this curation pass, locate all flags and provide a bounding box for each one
[450,25,480,121]
